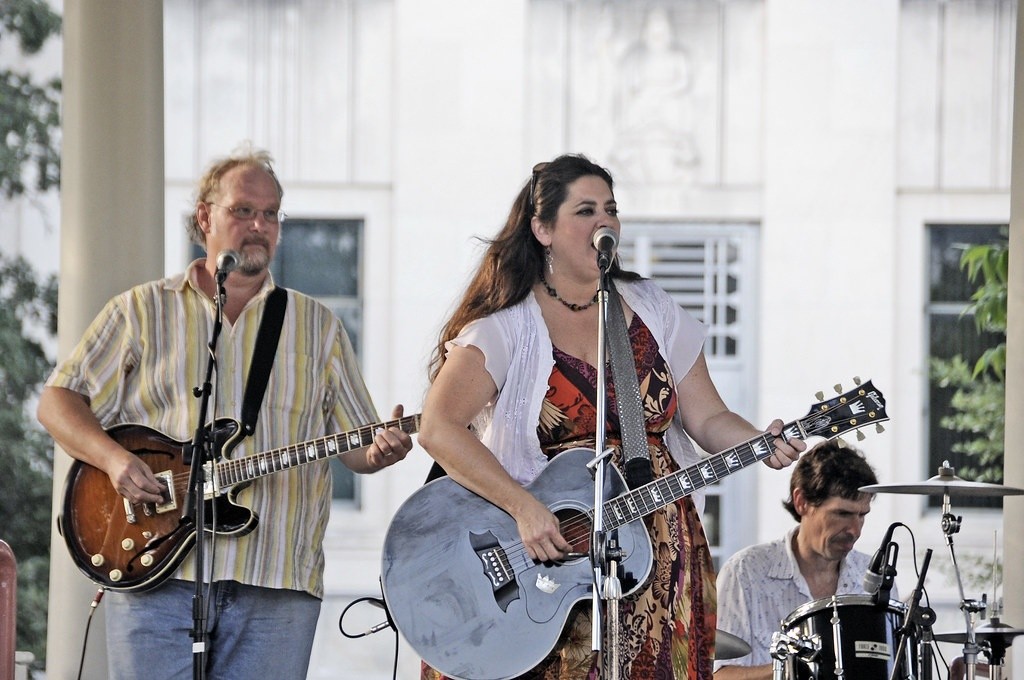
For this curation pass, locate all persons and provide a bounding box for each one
[714,440,898,680]
[417,152,807,680]
[38,157,413,680]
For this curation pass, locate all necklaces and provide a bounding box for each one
[538,267,598,312]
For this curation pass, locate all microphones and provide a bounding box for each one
[215,249,241,284]
[863,522,902,594]
[592,227,619,270]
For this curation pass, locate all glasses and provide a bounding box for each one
[207,202,288,223]
[531,162,549,207]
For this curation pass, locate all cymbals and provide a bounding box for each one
[714,628,753,661]
[854,473,1024,497]
[932,623,1024,643]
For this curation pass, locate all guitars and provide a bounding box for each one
[58,410,473,595]
[378,375,891,680]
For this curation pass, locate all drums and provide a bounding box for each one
[783,593,923,680]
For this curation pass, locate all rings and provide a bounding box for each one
[385,452,392,456]
